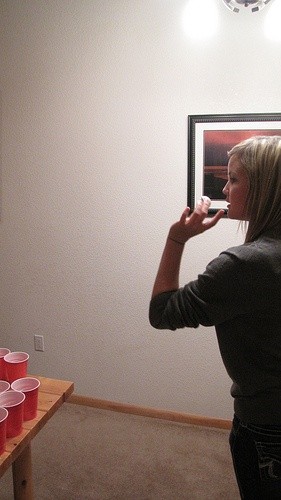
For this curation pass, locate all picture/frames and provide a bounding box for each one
[187,112,281,217]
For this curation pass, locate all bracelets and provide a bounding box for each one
[168,236,185,245]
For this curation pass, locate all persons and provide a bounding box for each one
[149,134,281,500]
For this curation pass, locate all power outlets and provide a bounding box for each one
[34,335,44,352]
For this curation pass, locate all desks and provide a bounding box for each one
[0,374,74,500]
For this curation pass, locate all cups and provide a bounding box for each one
[4,352,30,384]
[0,381,10,395]
[11,377,40,420]
[0,348,11,381]
[0,390,26,438]
[0,407,9,455]
